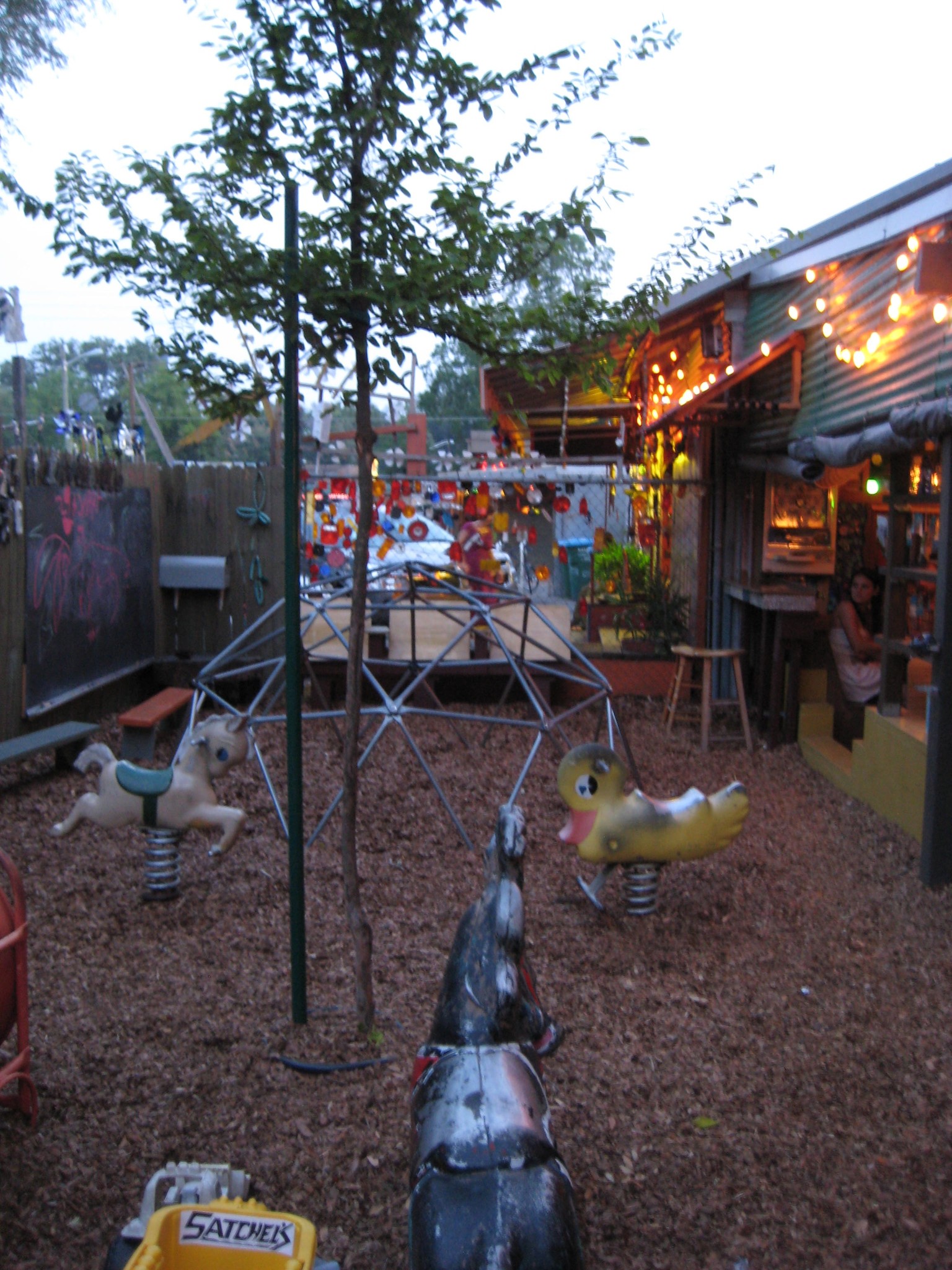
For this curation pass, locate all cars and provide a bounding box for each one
[300,501,515,590]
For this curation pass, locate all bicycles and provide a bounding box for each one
[510,546,539,594]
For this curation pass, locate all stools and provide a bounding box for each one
[663,645,752,754]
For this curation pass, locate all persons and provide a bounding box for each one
[457,507,503,627]
[571,532,631,628]
[831,566,883,703]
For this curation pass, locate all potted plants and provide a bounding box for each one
[586,536,690,661]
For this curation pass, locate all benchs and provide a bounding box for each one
[0,721,102,770]
[118,688,194,762]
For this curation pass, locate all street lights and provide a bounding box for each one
[130,363,146,427]
[63,347,105,411]
[402,345,416,414]
